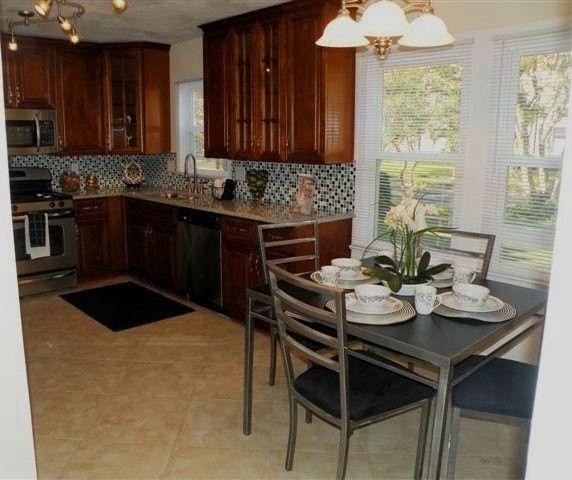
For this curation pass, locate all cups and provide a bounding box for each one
[413,284,442,315]
[453,267,476,283]
[314,266,339,287]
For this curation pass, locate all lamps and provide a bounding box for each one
[315,0,455,60]
[0,0,128,52]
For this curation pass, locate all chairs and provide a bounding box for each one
[265,261,437,480]
[258,220,367,423]
[414,227,497,279]
[445,354,540,479]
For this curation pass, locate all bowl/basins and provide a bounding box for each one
[331,258,362,278]
[354,285,391,310]
[451,283,489,306]
[414,257,448,273]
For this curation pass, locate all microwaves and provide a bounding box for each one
[6,109,59,156]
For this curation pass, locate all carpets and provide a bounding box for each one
[59,281,197,332]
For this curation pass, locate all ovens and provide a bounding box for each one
[11,210,79,298]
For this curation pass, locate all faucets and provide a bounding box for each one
[183,153,198,193]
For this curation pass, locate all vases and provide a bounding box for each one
[382,271,433,296]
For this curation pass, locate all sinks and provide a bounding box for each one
[152,188,192,201]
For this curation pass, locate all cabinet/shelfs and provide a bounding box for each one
[103,41,171,155]
[55,38,103,155]
[0,32,55,110]
[197,0,356,165]
[73,197,125,274]
[221,212,352,338]
[124,195,173,294]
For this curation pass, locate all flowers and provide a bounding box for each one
[359,187,452,293]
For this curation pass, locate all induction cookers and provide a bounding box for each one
[10,189,72,205]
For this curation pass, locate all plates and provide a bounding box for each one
[437,292,504,313]
[339,267,371,280]
[345,292,403,315]
